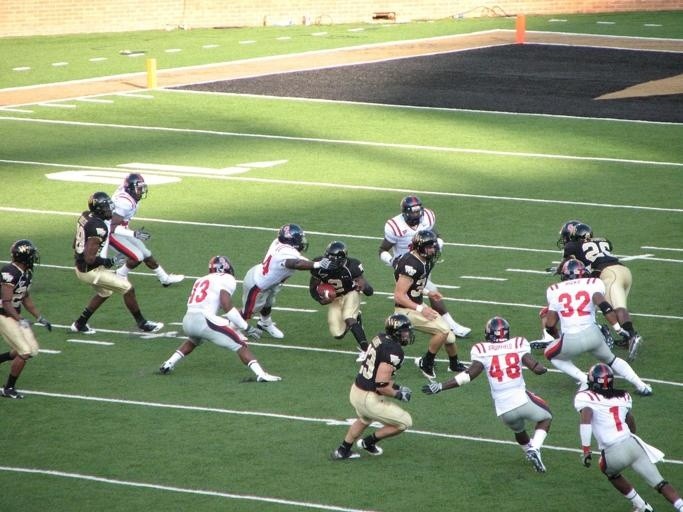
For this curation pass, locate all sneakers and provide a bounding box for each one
[357,438,383,457]
[159,361,173,374]
[161,273,185,289]
[139,320,164,334]
[70,321,96,337]
[633,501,654,512]
[637,384,652,396]
[330,448,361,460]
[256,373,282,384]
[627,333,643,363]
[413,356,438,383]
[444,363,468,375]
[525,448,547,476]
[528,337,554,348]
[256,319,284,340]
[452,324,472,338]
[0,386,23,400]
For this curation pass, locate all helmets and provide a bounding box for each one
[484,316,512,344]
[412,229,440,265]
[208,255,234,275]
[556,220,594,250]
[277,223,310,253]
[586,363,616,393]
[323,240,349,269]
[560,259,585,281]
[9,239,39,273]
[87,191,115,221]
[123,172,148,202]
[400,195,424,219]
[383,314,415,346]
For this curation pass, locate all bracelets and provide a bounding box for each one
[314,261,320,269]
[379,251,393,265]
[422,288,429,295]
[437,238,445,252]
[417,305,423,314]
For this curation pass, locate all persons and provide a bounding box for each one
[308,241,374,352]
[379,196,470,337]
[529,224,682,512]
[422,316,552,475]
[158,256,282,383]
[73,192,165,334]
[333,315,414,460]
[107,174,185,287]
[394,230,471,379]
[218,223,332,339]
[0,238,52,398]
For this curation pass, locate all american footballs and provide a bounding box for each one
[317,284,337,300]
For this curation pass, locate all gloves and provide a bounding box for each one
[109,252,126,267]
[36,316,52,332]
[580,452,592,469]
[396,384,412,402]
[246,325,262,338]
[420,381,442,396]
[18,318,34,328]
[132,226,151,242]
[617,327,631,343]
[319,257,337,271]
[351,278,365,292]
[319,295,333,306]
